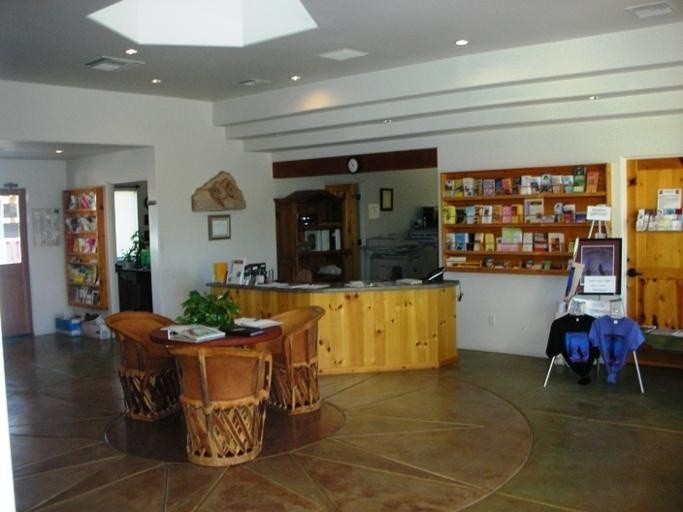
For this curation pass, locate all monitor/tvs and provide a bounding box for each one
[428,267,446,281]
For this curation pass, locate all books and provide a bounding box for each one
[65,190,102,307]
[158,317,285,344]
[443,167,601,271]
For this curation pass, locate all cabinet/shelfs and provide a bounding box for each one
[62,188,109,310]
[441,162,612,276]
[273,189,352,284]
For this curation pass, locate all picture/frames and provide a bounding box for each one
[380,188,394,212]
[576,237,621,296]
[209,215,231,240]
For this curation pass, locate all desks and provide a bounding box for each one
[115,261,153,312]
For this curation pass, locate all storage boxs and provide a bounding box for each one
[56,315,112,340]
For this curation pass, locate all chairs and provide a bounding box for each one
[106,304,325,467]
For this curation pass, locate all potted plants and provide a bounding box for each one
[121,251,133,271]
[129,230,146,269]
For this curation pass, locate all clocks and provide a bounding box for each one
[347,158,360,174]
